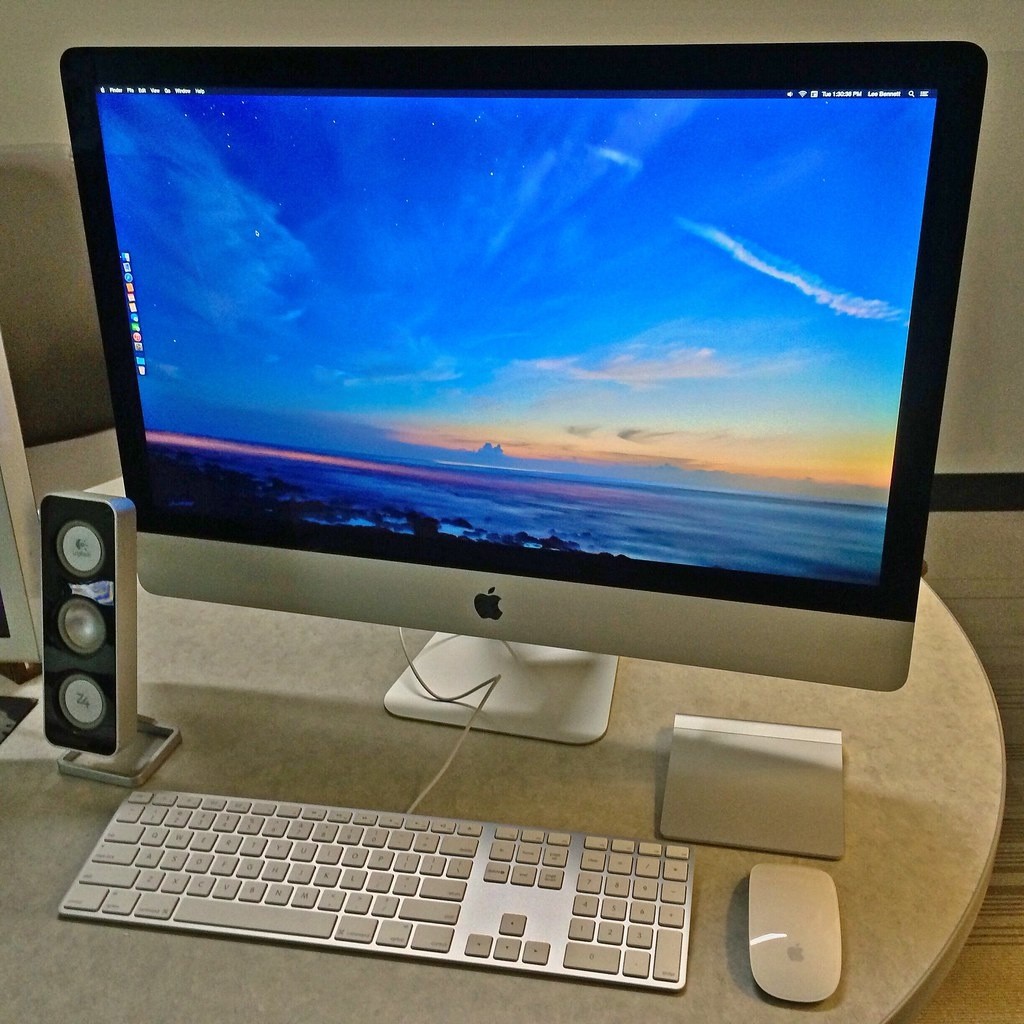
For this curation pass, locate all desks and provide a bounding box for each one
[1,476,1007,1022]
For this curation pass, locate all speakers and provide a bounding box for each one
[39,491,183,788]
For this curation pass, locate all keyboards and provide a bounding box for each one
[58,791,693,993]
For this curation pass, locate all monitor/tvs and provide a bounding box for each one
[59,41,986,743]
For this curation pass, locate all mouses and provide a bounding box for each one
[750,864,841,1002]
